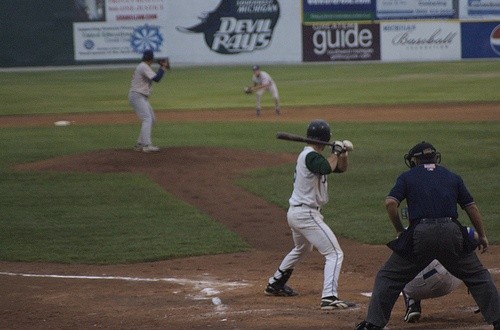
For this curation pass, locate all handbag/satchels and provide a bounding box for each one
[456,220,481,253]
[386,224,414,252]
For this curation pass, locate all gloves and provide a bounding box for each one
[342,140,353,153]
[331,140,344,156]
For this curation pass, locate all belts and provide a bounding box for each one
[417,216,454,224]
[293,205,319,210]
[423,269,437,280]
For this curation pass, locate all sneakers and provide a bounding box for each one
[320,297,356,310]
[264,283,299,297]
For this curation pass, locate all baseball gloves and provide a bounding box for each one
[243,86,252,95]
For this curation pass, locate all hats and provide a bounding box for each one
[144,50,154,57]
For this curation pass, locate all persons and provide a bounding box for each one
[127,49,167,152]
[247,64,281,116]
[264,120,356,310]
[355,141,500,330]
[401,227,480,323]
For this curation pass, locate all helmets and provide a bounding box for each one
[403,140,441,168]
[252,65,260,71]
[306,120,331,142]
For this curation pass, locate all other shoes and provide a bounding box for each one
[133,142,161,153]
[403,302,422,323]
[493,318,500,330]
[355,320,384,330]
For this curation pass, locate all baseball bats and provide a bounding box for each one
[275,131,334,146]
